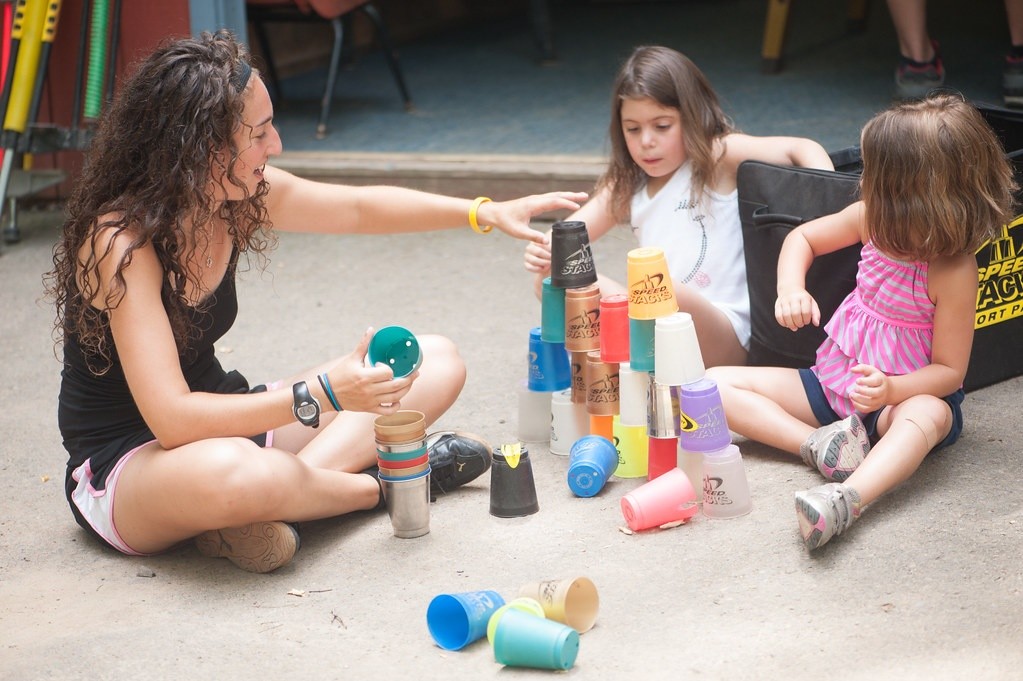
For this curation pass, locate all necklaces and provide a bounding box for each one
[196,221,214,268]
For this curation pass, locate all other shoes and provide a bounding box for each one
[1001,53,1023,106]
[894,37,946,96]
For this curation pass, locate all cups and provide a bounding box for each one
[369,327,423,377]
[515,221,752,519]
[621,467,698,530]
[488,448,539,519]
[374,410,432,539]
[428,576,600,671]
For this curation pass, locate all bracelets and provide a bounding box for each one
[317,373,343,411]
[470,196,494,234]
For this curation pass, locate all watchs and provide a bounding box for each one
[292,381,320,429]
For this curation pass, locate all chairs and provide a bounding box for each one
[248,0,415,140]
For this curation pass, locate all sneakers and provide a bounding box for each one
[793,482,861,551]
[194,520,303,574]
[426,430,492,494]
[799,414,871,484]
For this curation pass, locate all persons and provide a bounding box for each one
[41,31,589,574]
[704,96,1019,551]
[886,0,1023,107]
[522,45,836,368]
